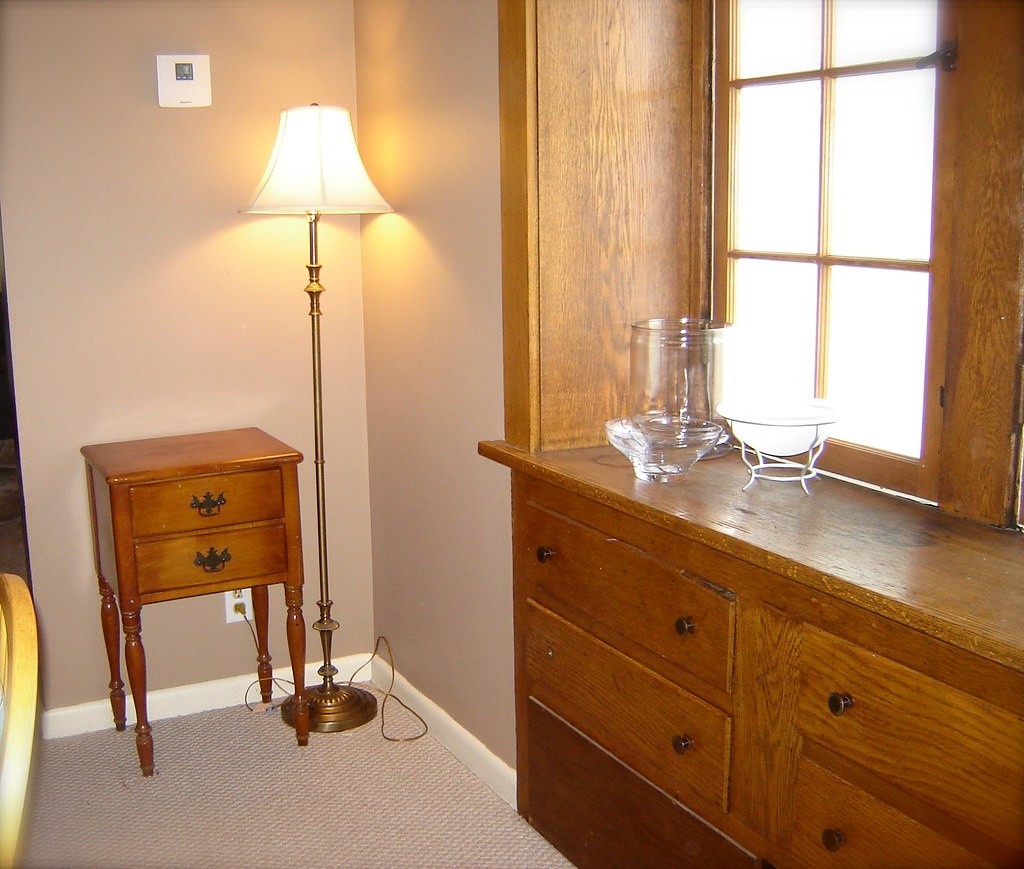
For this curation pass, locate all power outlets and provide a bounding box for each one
[225,588,252,623]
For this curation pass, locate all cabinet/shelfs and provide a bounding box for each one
[511,468,1024,869]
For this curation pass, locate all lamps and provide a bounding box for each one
[238,103,395,732]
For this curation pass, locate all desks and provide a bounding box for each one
[80,427,306,776]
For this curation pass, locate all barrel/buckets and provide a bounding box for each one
[629,318,734,458]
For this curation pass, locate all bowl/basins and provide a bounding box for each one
[604,415,723,483]
[715,396,842,456]
